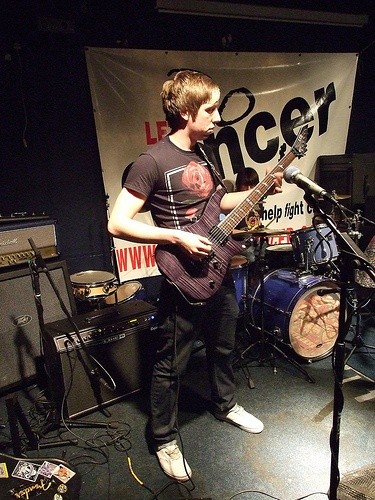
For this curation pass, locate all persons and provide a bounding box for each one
[107,70,283,481]
[225,167,267,263]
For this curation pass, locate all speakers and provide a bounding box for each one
[0,255,78,394]
[43,299,159,421]
[283,166,340,205]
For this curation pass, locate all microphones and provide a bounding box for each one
[91,373,111,390]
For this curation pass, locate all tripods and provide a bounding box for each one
[344,306,375,365]
[230,235,317,384]
[21,238,119,453]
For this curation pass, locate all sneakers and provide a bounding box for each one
[215,402,264,433]
[154,439,192,481]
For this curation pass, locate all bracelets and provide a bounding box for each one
[261,194,267,199]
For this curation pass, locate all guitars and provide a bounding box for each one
[155,124,315,301]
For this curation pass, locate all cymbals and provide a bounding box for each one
[311,192,353,201]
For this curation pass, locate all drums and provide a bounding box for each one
[252,268,343,362]
[69,270,118,299]
[106,281,148,306]
[291,223,340,269]
[266,244,298,266]
[228,255,250,304]
